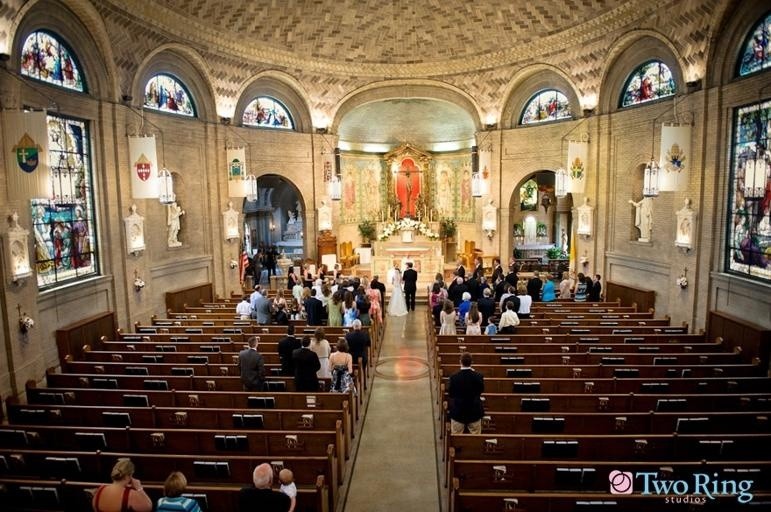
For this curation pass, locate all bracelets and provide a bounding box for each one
[137,487,143,492]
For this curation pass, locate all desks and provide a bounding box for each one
[514,258,538,264]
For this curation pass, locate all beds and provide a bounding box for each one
[548,259,570,271]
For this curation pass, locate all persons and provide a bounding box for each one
[157,471,203,512]
[92,460,152,511]
[278,319,371,393]
[253,241,280,286]
[429,258,533,335]
[387,264,408,317]
[278,468,297,512]
[33,204,90,272]
[240,337,264,391]
[236,264,385,327]
[561,229,567,257]
[237,462,291,512]
[403,262,417,312]
[447,352,484,434]
[527,270,601,302]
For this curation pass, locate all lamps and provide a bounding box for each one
[743,82,771,201]
[221,121,258,205]
[642,87,700,198]
[317,130,344,202]
[123,101,177,205]
[469,128,494,198]
[552,105,596,197]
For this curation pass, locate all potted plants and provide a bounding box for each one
[440,217,457,243]
[357,219,378,248]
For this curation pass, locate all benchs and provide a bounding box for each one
[135,277,389,357]
[423,290,672,338]
